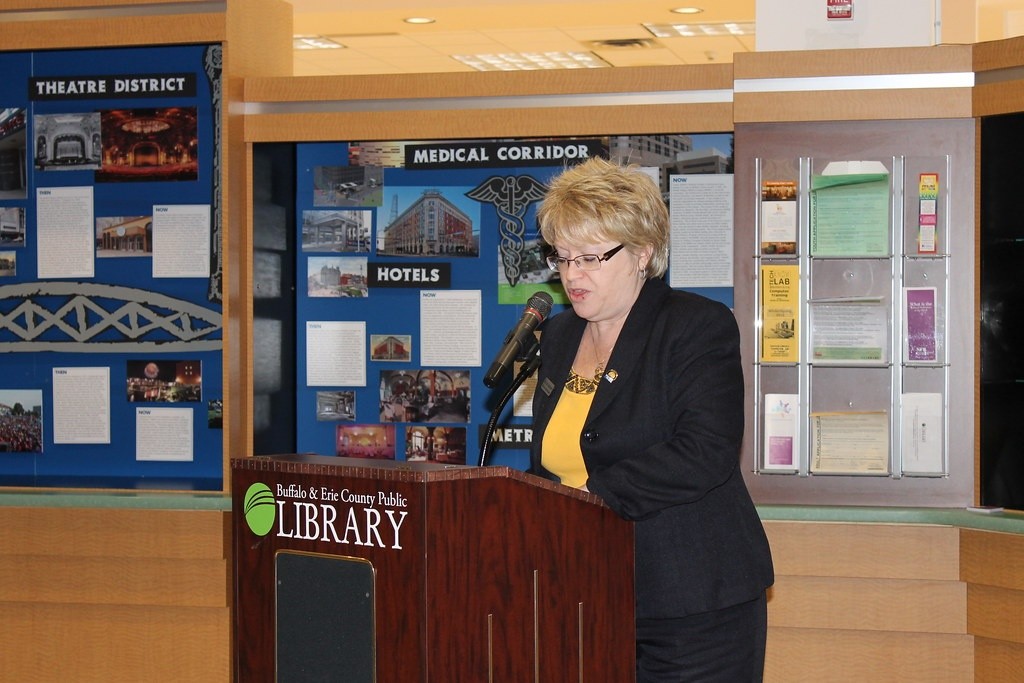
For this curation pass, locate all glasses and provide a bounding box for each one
[544,244,625,272]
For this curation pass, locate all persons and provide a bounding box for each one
[526,156,775,683]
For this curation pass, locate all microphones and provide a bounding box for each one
[483,291,553,389]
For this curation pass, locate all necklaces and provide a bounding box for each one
[589,321,613,375]
